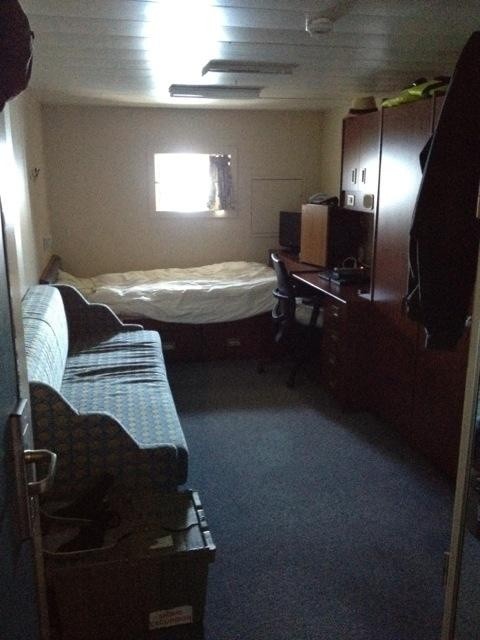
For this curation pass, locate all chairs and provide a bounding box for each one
[256,252,326,390]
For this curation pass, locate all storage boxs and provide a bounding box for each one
[30,487,219,640]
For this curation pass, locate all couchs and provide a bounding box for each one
[17,282,189,491]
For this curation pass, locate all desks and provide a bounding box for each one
[275,252,375,390]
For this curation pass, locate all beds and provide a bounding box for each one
[38,254,277,329]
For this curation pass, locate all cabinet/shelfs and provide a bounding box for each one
[335,88,480,480]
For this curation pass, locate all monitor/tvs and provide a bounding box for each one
[279,210,301,255]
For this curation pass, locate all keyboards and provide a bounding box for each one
[319,268,348,286]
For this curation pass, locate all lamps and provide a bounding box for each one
[199,56,301,79]
[166,82,266,102]
